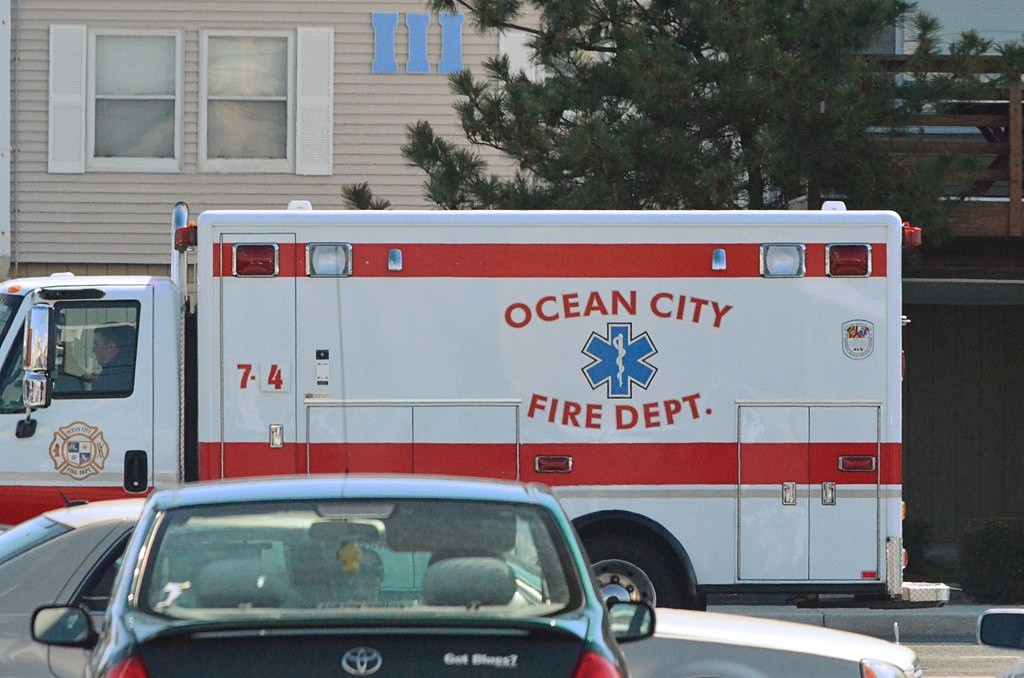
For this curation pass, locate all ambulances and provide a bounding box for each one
[1,200,961,613]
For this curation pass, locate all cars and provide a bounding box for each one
[0,473,924,677]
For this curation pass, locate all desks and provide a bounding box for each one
[904,102,1024,195]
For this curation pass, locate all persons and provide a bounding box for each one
[86,321,136,392]
[337,547,389,604]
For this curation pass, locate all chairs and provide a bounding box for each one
[297,542,385,606]
[419,543,518,606]
[197,557,288,610]
